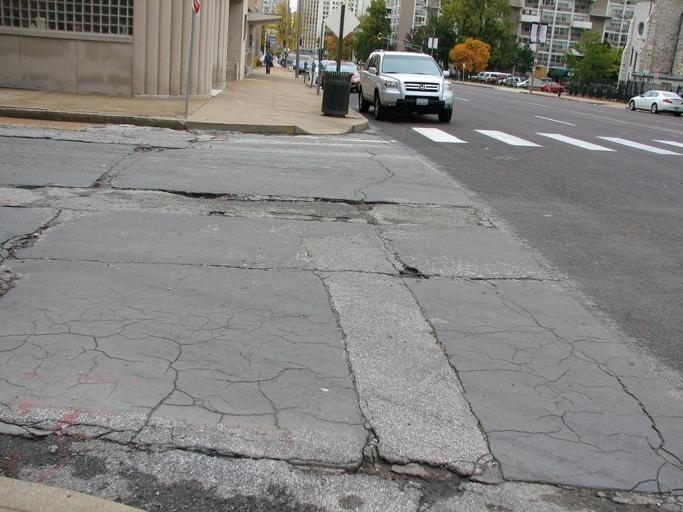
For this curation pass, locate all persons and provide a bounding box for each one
[263,52,273,74]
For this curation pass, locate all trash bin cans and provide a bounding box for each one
[322,71,354,117]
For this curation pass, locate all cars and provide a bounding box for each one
[278,48,454,123]
[469,71,565,94]
[627,89,682,116]
